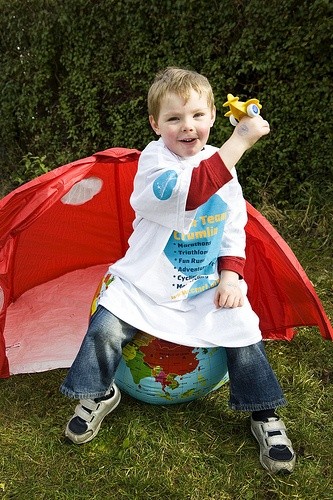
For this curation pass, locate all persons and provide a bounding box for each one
[59,67,295,476]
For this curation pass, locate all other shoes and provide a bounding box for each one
[64,381,122,444]
[250,413,295,474]
